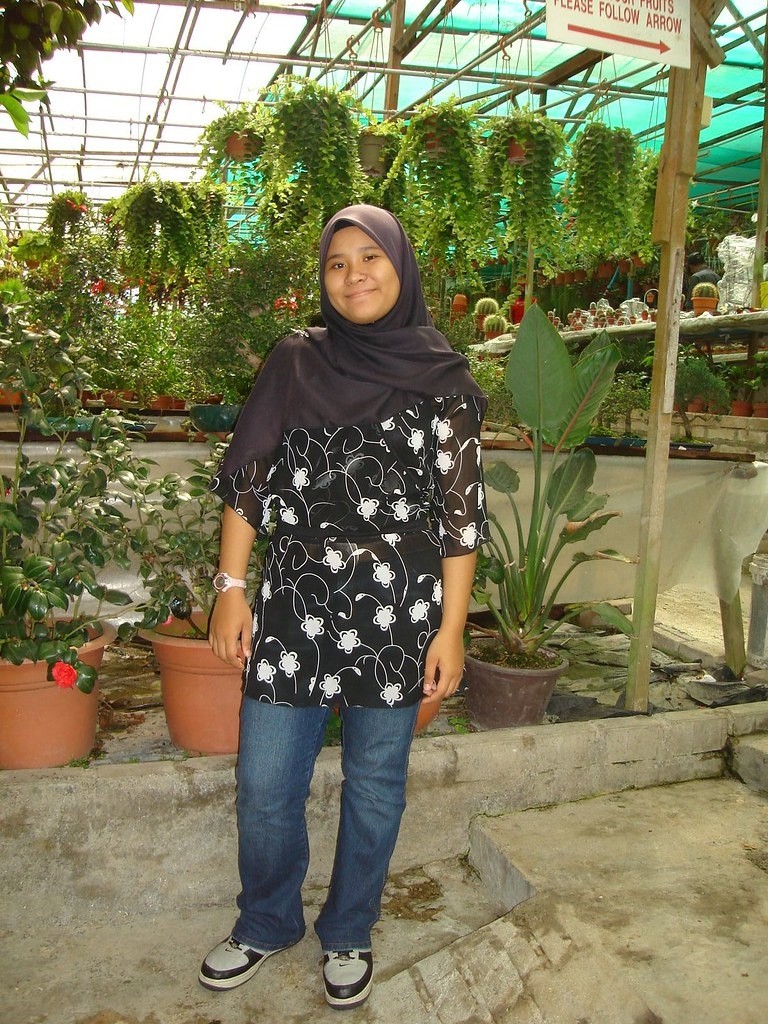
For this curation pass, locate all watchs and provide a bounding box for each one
[212,572,247,593]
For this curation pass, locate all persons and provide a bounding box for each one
[196,213,491,1006]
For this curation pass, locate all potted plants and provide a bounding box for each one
[473,295,499,332]
[560,121,660,267]
[463,301,640,728]
[601,369,650,447]
[759,270,768,310]
[169,366,191,409]
[103,362,137,408]
[728,365,752,416]
[583,407,619,446]
[648,355,732,452]
[146,367,172,410]
[358,114,406,212]
[76,361,106,406]
[479,102,568,278]
[190,366,255,432]
[548,292,657,331]
[752,379,768,418]
[377,91,501,293]
[482,313,509,340]
[195,99,294,219]
[690,282,720,317]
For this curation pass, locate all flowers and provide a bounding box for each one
[0,288,161,694]
[130,432,278,638]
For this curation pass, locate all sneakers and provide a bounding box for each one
[321,949,373,1009]
[198,934,291,992]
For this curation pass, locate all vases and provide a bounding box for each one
[137,611,244,753]
[574,269,586,282]
[631,254,646,269]
[687,389,706,413]
[0,616,118,770]
[598,263,616,280]
[564,271,574,283]
[708,400,727,415]
[618,258,630,273]
[0,386,32,406]
[498,255,507,265]
[555,273,564,285]
